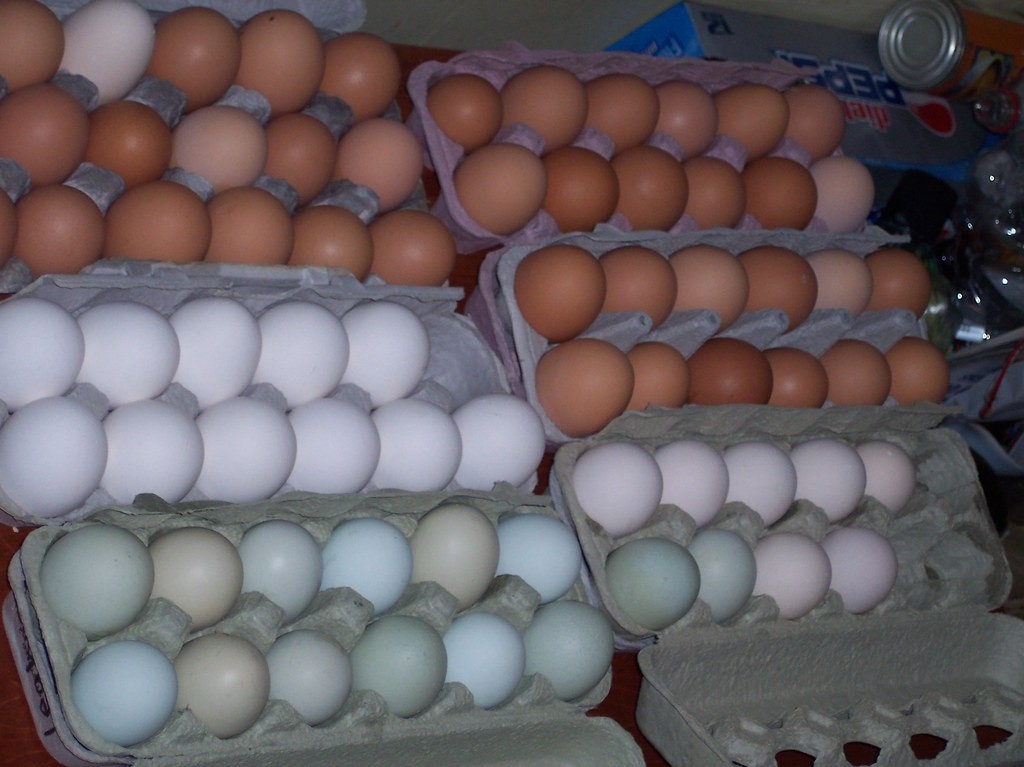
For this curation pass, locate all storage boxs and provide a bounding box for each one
[596,0,1002,224]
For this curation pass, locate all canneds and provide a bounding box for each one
[879,0,1024,101]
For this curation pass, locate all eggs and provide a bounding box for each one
[426,66,949,633]
[0,0,615,746]
